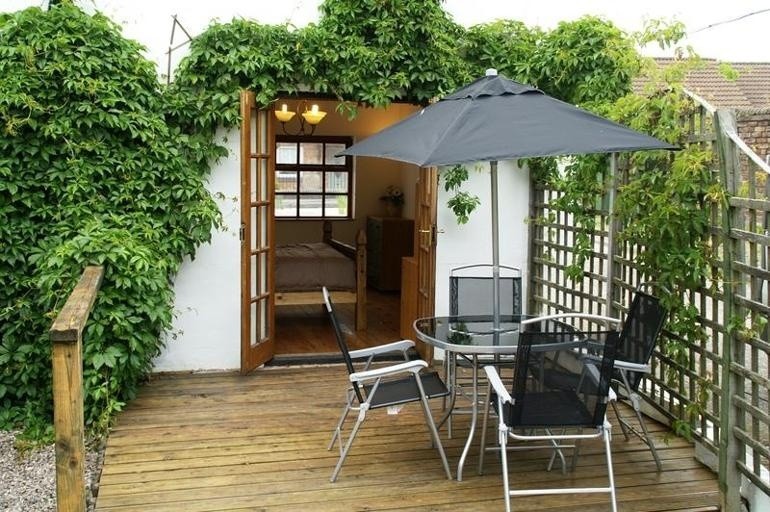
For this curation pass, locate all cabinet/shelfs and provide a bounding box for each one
[367,214,416,293]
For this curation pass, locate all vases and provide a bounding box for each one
[386,202,398,216]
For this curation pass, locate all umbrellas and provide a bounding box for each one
[333,67,684,381]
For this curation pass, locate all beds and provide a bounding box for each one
[276,222,369,332]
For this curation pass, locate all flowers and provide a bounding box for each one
[381,186,405,206]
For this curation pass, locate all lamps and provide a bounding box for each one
[273,98,326,138]
